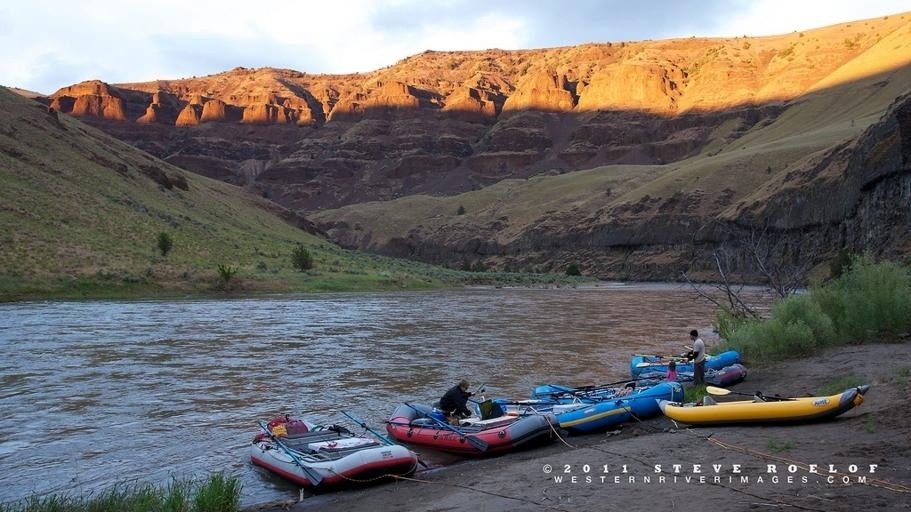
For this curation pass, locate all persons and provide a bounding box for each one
[666,361,677,382]
[438,380,476,418]
[679,329,705,385]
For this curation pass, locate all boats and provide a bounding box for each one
[656,384,872,425]
[251,413,421,492]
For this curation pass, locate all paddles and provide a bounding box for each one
[257,421,324,486]
[404,402,488,453]
[340,410,427,469]
[705,386,796,401]
